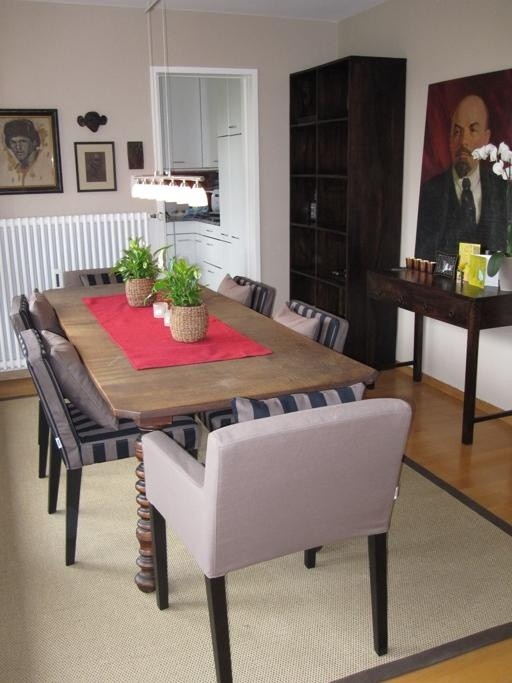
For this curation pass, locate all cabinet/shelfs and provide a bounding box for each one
[167,216,197,279]
[288,55,407,368]
[216,78,248,227]
[196,219,249,293]
[160,77,218,172]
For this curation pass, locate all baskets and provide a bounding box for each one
[125,279,156,307]
[171,303,207,342]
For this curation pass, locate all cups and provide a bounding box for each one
[405,257,436,272]
[130,178,209,207]
[405,269,433,285]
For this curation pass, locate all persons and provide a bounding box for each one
[1,119,56,188]
[415,92,512,288]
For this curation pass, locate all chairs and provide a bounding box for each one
[142,397,410,682]
[288,296,350,352]
[8,292,74,480]
[219,274,275,317]
[62,265,124,287]
[16,326,203,568]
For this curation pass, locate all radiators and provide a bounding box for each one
[1,210,166,371]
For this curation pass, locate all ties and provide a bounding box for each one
[460,179,476,238]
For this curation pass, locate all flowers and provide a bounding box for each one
[470,140,512,277]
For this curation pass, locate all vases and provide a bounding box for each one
[498,255,511,292]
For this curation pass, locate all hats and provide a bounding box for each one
[4,119,40,148]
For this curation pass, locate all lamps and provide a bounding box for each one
[128,1,210,207]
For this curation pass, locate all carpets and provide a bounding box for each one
[2,394,510,681]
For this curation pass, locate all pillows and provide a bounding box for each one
[231,382,366,423]
[37,327,121,433]
[215,273,253,308]
[29,286,65,334]
[80,269,124,286]
[272,303,321,339]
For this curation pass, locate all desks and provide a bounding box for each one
[362,266,511,444]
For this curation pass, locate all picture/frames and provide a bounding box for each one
[74,140,117,191]
[433,250,458,279]
[1,107,64,194]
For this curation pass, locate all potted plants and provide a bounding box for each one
[119,235,172,308]
[154,259,210,343]
